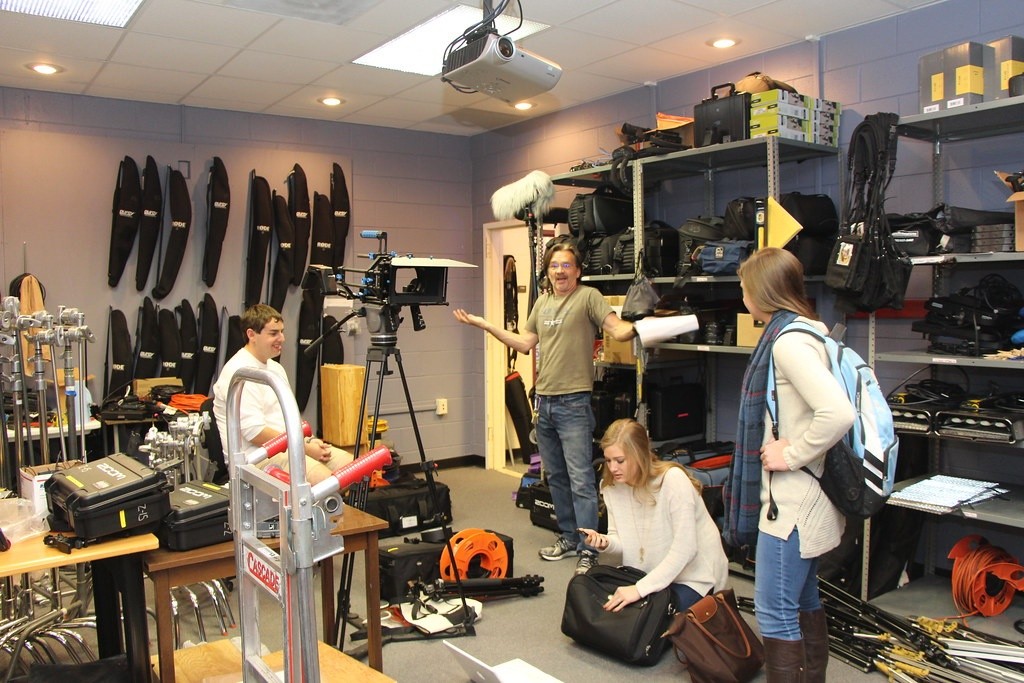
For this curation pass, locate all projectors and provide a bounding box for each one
[442,34,565,106]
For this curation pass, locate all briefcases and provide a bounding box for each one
[591,375,706,443]
[692,83,752,147]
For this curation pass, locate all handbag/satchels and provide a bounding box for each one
[826,231,912,309]
[565,183,675,276]
[670,589,766,682]
[782,190,837,273]
[354,479,452,540]
[560,560,674,661]
[378,529,515,600]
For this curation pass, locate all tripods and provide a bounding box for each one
[305,303,476,661]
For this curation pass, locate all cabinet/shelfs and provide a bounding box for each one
[536,134,847,563]
[861,95,1024,647]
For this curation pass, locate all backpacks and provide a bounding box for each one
[677,192,768,270]
[766,320,898,517]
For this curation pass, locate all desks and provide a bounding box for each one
[0,415,398,683]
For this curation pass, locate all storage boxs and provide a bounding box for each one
[750,102,809,121]
[151,479,235,553]
[43,453,175,539]
[750,125,808,142]
[750,89,810,109]
[983,34,1024,102]
[993,170,1024,252]
[590,367,706,442]
[750,115,808,133]
[809,97,842,116]
[808,121,839,138]
[19,459,84,516]
[133,377,186,402]
[602,295,638,364]
[917,41,984,114]
[808,133,838,148]
[35,367,95,413]
[809,110,840,126]
[737,313,767,348]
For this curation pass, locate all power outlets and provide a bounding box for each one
[436,399,448,415]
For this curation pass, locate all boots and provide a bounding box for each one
[799,606,828,682]
[762,632,805,682]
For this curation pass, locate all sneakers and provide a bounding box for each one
[538,533,581,561]
[574,549,600,577]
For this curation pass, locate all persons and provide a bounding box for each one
[578,420,729,610]
[722,248,856,683]
[452,244,657,575]
[212,304,355,483]
